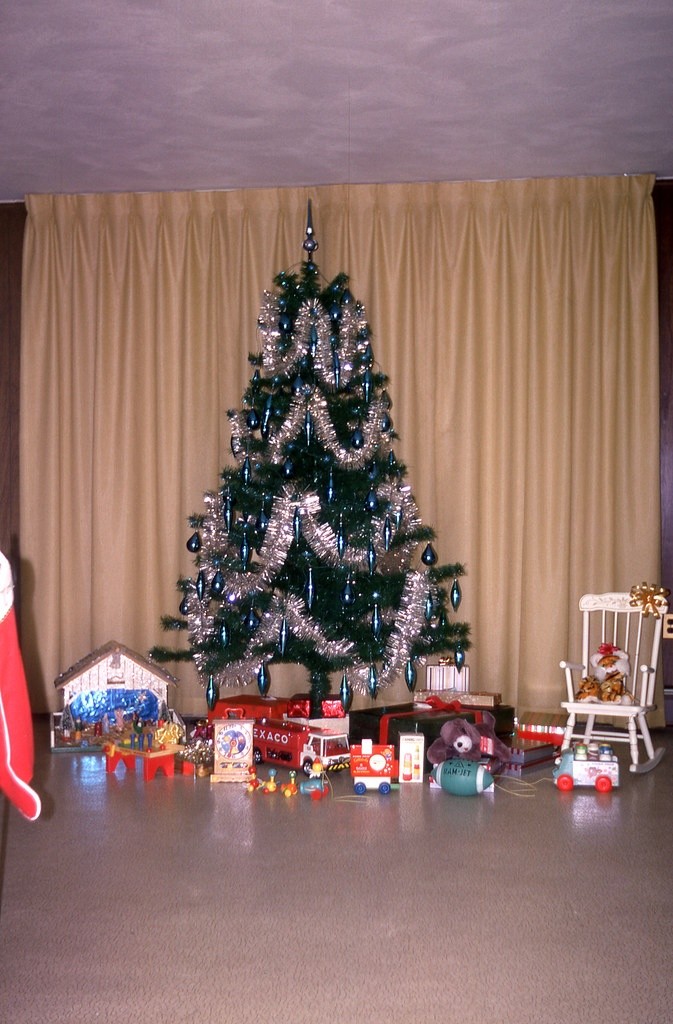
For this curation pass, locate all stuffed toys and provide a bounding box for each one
[569,642,641,705]
[427,711,511,763]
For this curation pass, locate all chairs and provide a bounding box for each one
[551,591,669,775]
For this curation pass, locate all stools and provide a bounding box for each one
[98,739,186,783]
